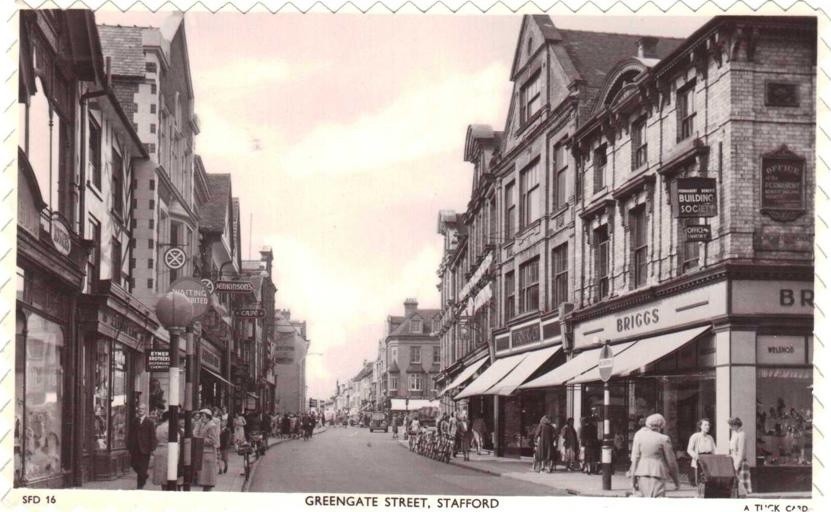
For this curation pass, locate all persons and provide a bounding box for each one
[391,411,487,461]
[686,418,719,484]
[727,417,752,498]
[630,413,680,497]
[531,414,598,474]
[126,402,325,492]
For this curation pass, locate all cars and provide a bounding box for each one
[367,410,388,433]
[346,413,360,426]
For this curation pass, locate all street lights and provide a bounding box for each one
[154,289,193,492]
[296,350,324,413]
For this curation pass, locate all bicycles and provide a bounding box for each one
[405,429,455,465]
[232,438,254,481]
[247,428,267,459]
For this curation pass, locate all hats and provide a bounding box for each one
[644,412,666,432]
[198,408,213,416]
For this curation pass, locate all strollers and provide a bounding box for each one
[694,452,744,498]
[531,436,558,472]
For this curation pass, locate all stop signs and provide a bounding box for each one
[598,344,614,383]
